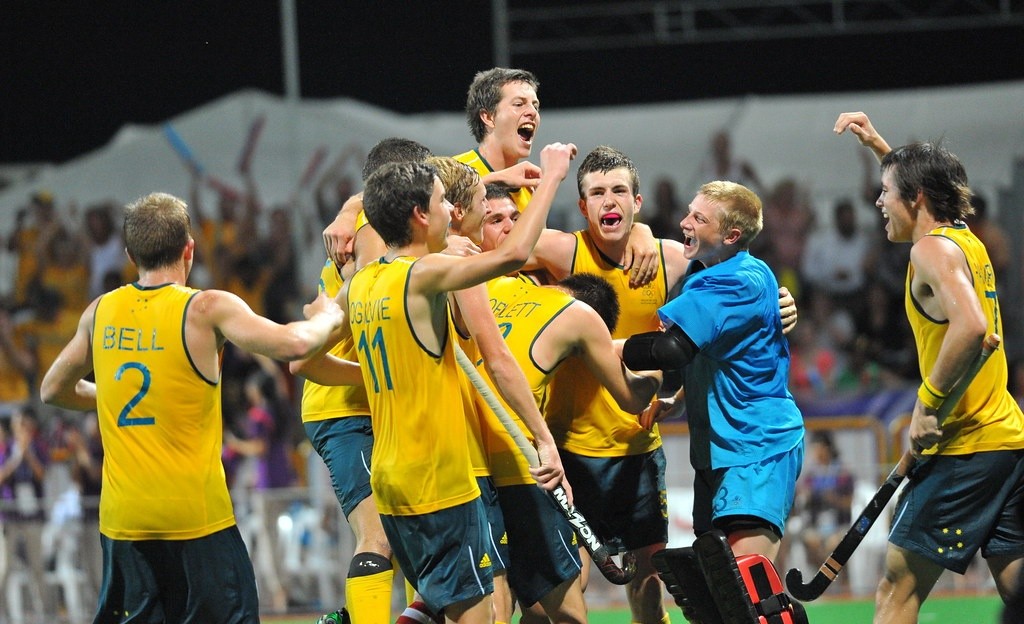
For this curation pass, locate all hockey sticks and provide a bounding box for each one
[453,341,638,584]
[786,333,1002,602]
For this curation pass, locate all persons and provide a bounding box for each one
[834,112,1024,624]
[0,61,1024,624]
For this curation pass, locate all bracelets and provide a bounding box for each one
[918,377,947,411]
